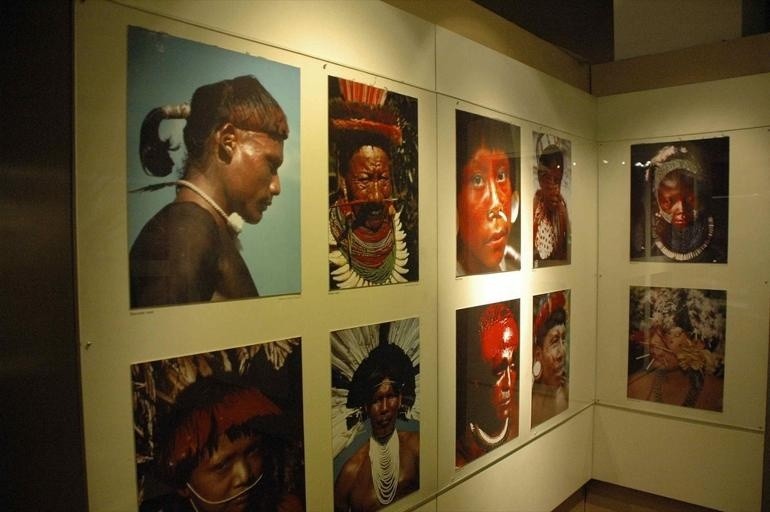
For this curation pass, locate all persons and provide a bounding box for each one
[633,143,729,263]
[455,109,522,281]
[627,286,725,414]
[528,293,575,429]
[138,371,307,511]
[128,73,291,314]
[533,133,572,268]
[333,374,422,512]
[328,137,417,293]
[456,300,522,469]
